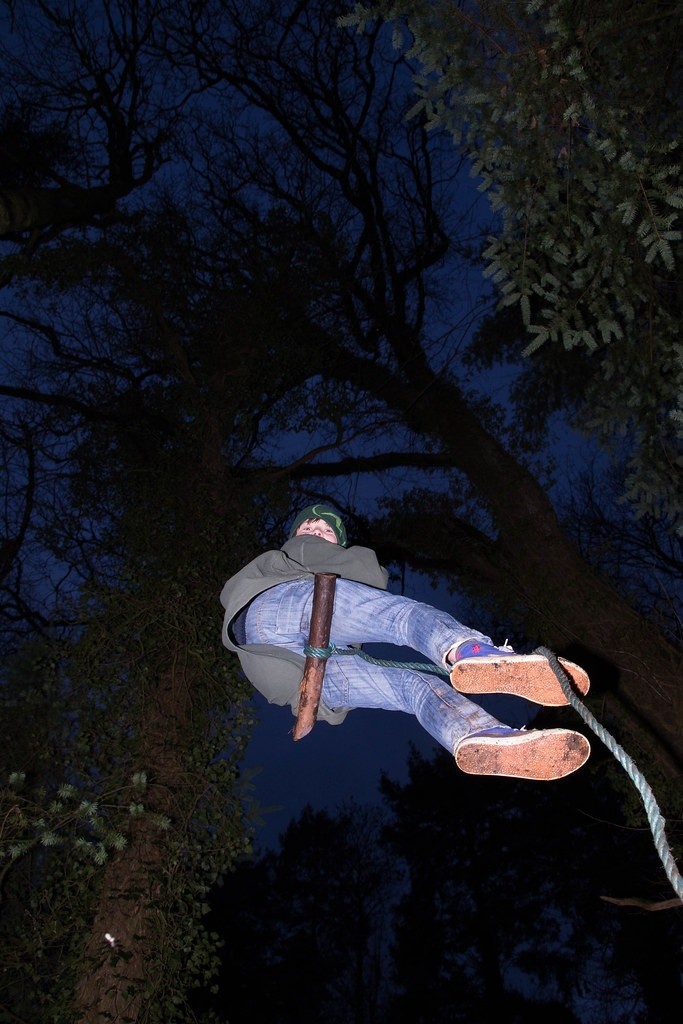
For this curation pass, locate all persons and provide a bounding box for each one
[220,505,590,781]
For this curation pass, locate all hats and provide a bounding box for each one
[289,504,348,547]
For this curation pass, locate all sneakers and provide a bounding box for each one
[453,724,590,781]
[446,641,592,708]
